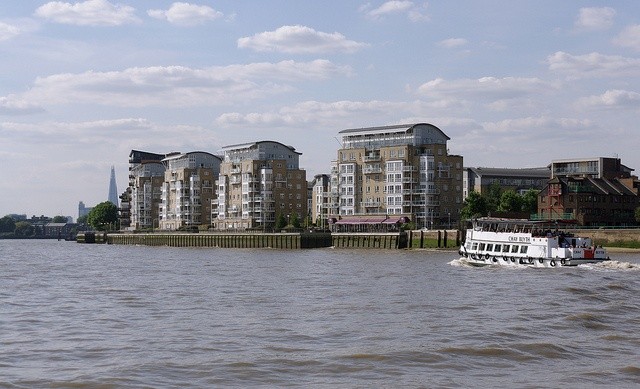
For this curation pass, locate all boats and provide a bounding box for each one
[456,215,612,269]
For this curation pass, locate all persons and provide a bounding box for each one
[485,226,604,250]
